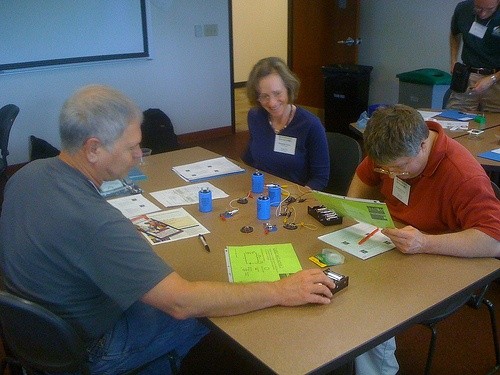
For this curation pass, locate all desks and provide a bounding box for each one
[349,107,500,180]
[98,145,500,375]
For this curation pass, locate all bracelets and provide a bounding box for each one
[490,74,496,84]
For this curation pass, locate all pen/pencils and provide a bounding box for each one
[359,228,378,245]
[199,234,210,252]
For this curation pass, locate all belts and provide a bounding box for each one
[471,68,500,75]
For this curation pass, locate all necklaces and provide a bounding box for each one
[269,104,292,134]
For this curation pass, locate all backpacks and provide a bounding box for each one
[140,109,178,155]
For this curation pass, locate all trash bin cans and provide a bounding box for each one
[397,68,452,109]
[322,64,372,137]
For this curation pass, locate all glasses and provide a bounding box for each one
[473,4,497,14]
[256,86,284,102]
[373,153,415,176]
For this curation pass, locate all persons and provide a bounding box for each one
[239,57,330,192]
[0,84,335,375]
[345,103,500,375]
[444,0,500,112]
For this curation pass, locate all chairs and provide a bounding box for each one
[0,290,179,375]
[415,180,500,375]
[321,131,363,196]
[0,104,20,181]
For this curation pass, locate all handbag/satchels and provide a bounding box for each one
[450,62,471,93]
